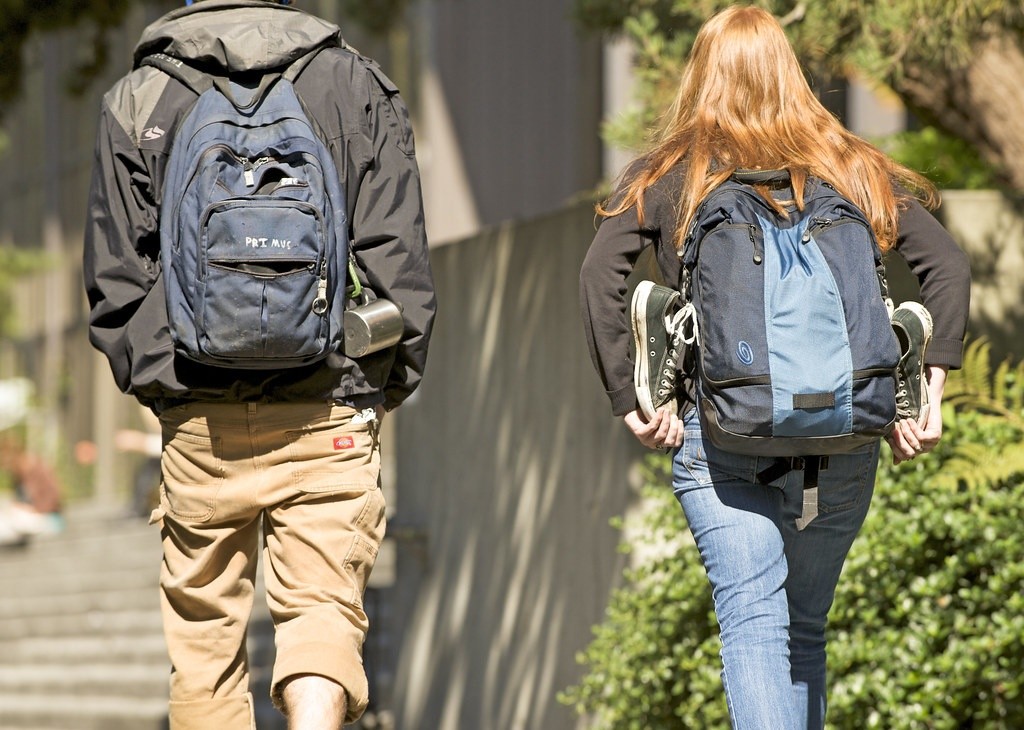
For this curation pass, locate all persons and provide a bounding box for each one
[83,0,436,729]
[580,5,972,729]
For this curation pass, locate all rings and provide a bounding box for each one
[655,444,663,450]
[914,441,925,452]
[906,456,916,461]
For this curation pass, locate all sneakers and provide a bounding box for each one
[891,301,934,431]
[631,279,699,422]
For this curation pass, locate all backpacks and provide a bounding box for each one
[142,46,358,372]
[679,170,903,529]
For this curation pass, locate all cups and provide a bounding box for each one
[346,287,404,357]
